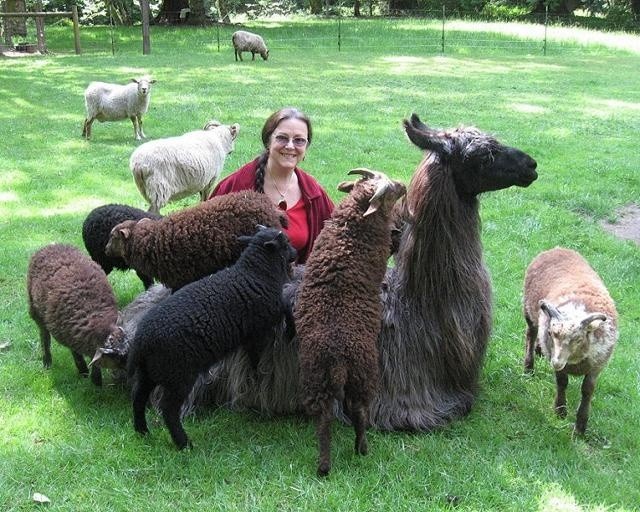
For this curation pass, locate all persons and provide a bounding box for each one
[205,106,336,264]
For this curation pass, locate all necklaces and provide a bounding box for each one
[266,163,295,205]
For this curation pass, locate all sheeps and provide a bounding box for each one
[117,110,541,437]
[128,119,241,214]
[521,243,620,441]
[25,241,136,391]
[81,76,158,142]
[231,29,271,62]
[103,185,291,298]
[80,202,183,293]
[294,167,410,480]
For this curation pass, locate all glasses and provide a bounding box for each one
[271,134,308,147]
